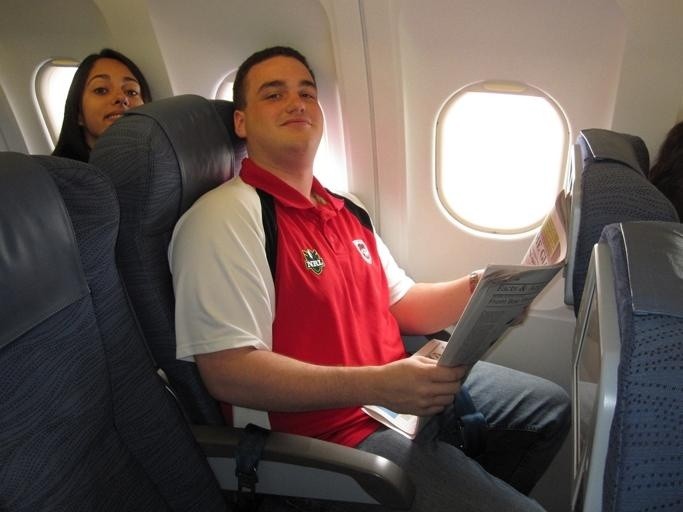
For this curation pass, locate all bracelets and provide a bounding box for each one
[469,271,478,293]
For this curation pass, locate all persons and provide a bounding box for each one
[165,44,570,512]
[51,48,153,164]
[646,117,683,224]
